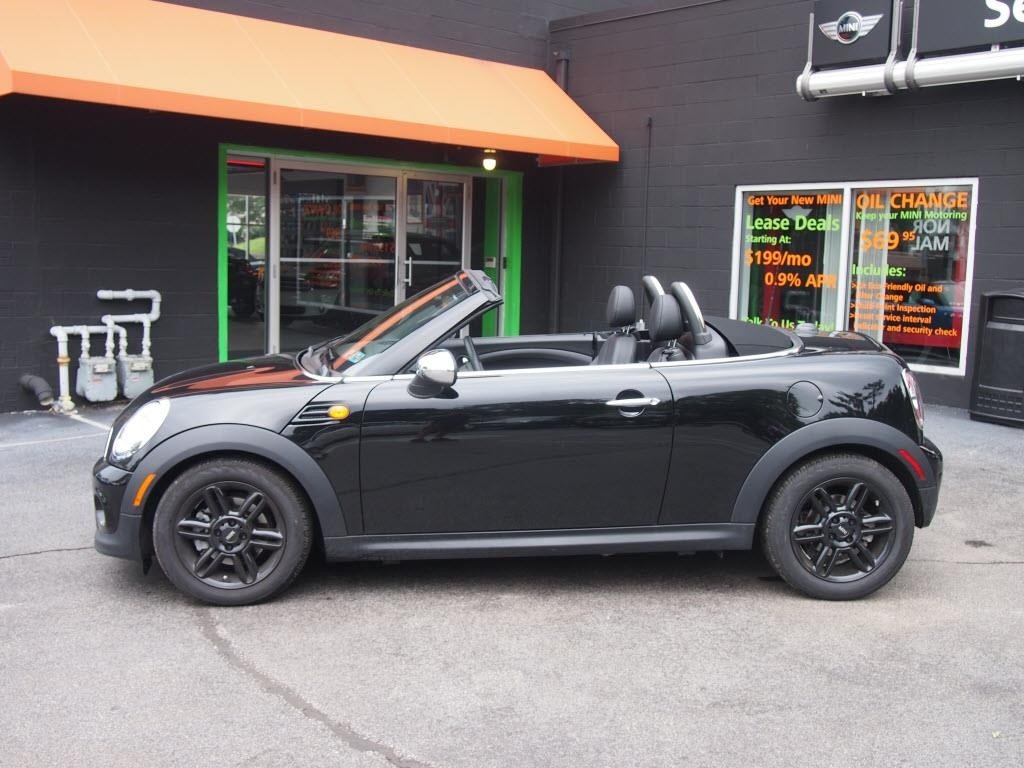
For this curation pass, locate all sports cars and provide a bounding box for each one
[92,270,945,610]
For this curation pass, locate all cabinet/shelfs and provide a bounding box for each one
[280,194,464,312]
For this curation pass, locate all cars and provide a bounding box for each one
[358,231,484,307]
[914,278,966,355]
[228,246,310,323]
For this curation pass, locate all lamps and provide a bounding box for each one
[483,148,497,171]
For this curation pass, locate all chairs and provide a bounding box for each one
[587,285,685,365]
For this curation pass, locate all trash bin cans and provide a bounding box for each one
[969,289,1024,428]
